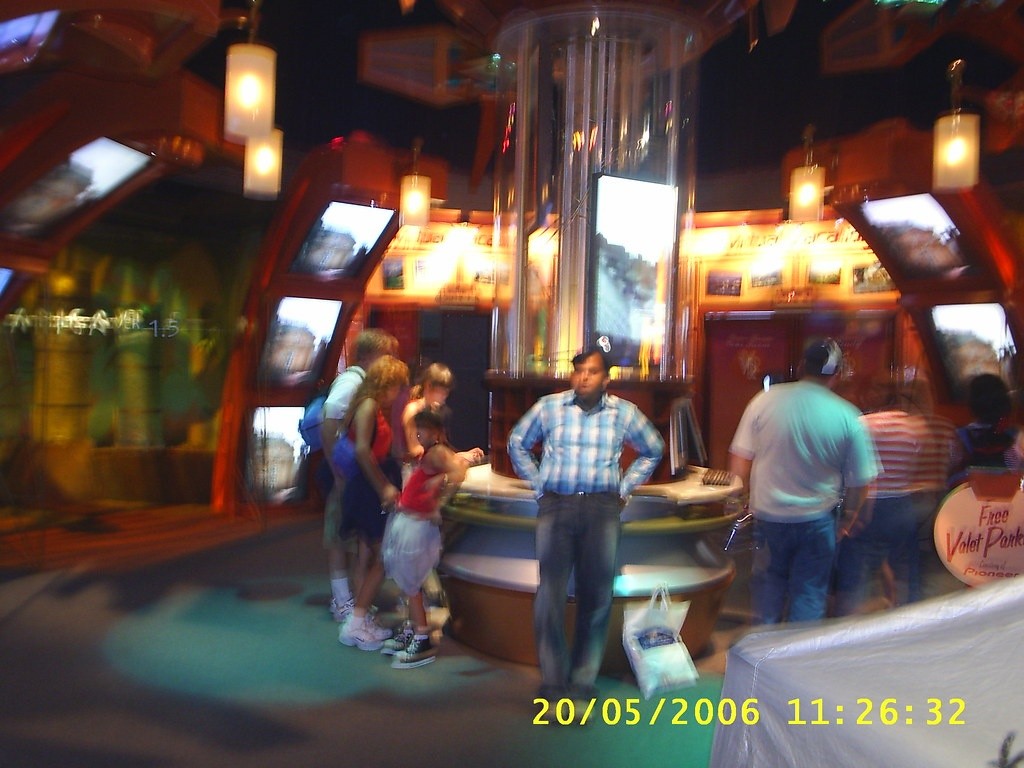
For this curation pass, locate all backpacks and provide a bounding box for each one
[297,369,364,460]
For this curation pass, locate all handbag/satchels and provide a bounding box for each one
[622,585,701,700]
[325,401,378,492]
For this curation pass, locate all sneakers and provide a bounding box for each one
[363,621,391,641]
[381,628,413,654]
[388,637,437,670]
[328,593,376,625]
[338,619,382,651]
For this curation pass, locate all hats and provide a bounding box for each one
[801,336,842,376]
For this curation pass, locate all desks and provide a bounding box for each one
[440,465,744,535]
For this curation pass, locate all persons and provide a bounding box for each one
[508,347,665,721]
[323,329,484,668]
[726,336,1023,624]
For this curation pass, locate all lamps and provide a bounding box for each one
[933,53,981,191]
[219,1,278,143]
[790,158,825,231]
[398,139,433,228]
[242,129,283,202]
